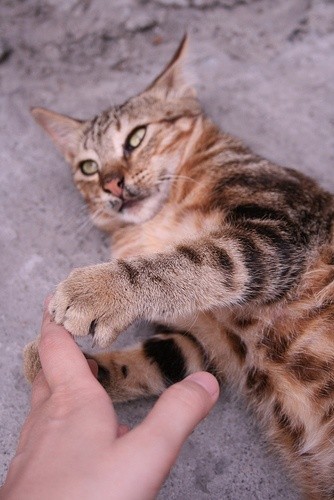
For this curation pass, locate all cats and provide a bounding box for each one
[18,30,334,500]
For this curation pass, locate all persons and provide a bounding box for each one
[0,292,222,500]
